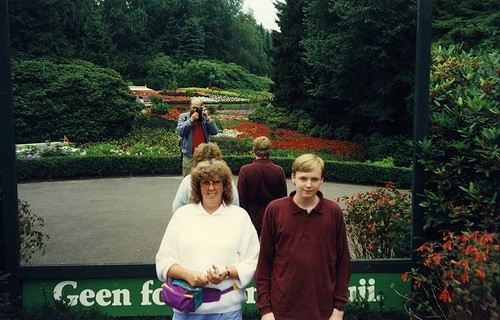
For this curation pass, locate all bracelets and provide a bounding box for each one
[226,267,230,279]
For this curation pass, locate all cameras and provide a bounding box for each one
[194,108,202,115]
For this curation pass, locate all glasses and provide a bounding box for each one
[200,180,223,188]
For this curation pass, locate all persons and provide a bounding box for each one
[236,137,287,238]
[171,143,240,212]
[155,159,260,320]
[255,153,351,320]
[177,98,219,179]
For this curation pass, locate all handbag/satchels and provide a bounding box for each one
[161,276,238,314]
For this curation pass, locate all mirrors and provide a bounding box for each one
[0,0,433,283]
